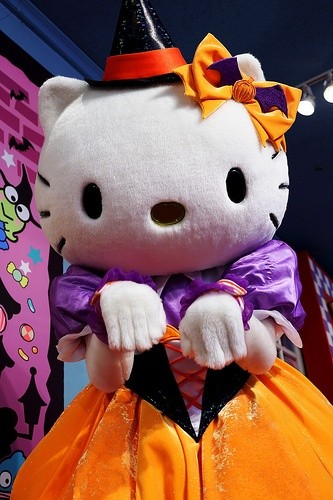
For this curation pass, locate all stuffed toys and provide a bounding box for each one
[9,1,332,500]
